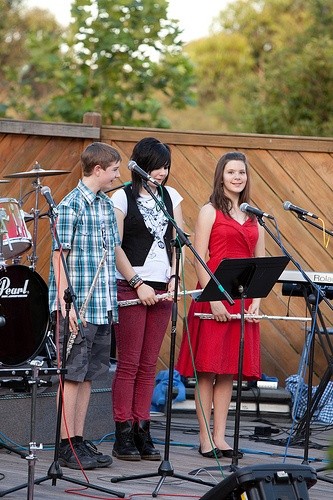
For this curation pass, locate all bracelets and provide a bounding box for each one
[129,274,143,289]
[169,275,180,281]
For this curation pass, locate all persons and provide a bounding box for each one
[174,151,265,460]
[47,143,121,470]
[111,137,186,460]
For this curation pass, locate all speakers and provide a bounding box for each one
[199,464,317,500]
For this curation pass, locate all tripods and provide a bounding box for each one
[188,255,291,483]
[255,210,332,484]
[1,202,125,499]
[110,172,235,498]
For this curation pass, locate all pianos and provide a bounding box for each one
[276,270,333,300]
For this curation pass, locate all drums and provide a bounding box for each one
[0,263,51,367]
[0,197,33,260]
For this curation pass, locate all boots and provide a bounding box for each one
[114,421,141,460]
[136,422,161,460]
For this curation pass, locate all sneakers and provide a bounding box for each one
[78,440,113,469]
[57,441,96,469]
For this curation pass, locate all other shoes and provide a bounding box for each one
[199,444,222,458]
[219,448,243,458]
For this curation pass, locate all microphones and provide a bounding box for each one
[239,203,275,220]
[128,161,161,187]
[283,200,320,219]
[40,186,58,216]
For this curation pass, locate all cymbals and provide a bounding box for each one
[2,168,71,178]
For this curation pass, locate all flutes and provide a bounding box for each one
[118,288,205,307]
[58,250,107,369]
[194,312,312,322]
